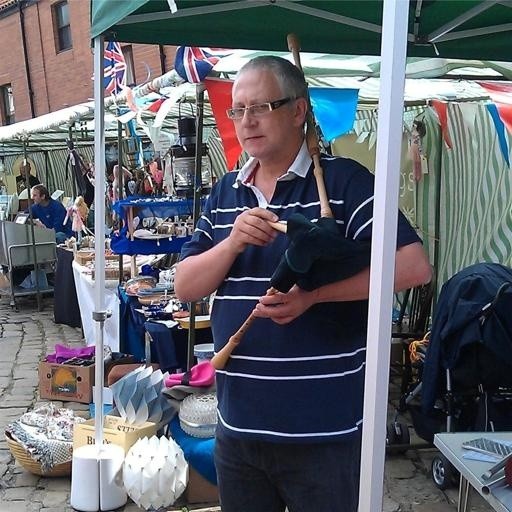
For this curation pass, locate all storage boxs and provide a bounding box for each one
[105,352,134,387]
[169,417,219,504]
[72,415,156,458]
[38,353,111,404]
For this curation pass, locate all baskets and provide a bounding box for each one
[3,431,71,477]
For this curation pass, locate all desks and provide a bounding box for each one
[433,430,512,512]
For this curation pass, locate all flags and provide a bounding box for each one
[175,45,232,84]
[90,40,126,97]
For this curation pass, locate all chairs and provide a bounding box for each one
[393,264,437,414]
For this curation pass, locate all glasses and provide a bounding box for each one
[226,97,291,120]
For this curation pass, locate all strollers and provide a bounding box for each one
[385,260,512,494]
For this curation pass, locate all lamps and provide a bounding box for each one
[167,100,214,196]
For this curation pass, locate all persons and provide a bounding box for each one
[172,56,436,511]
[16,162,41,193]
[22,186,72,247]
